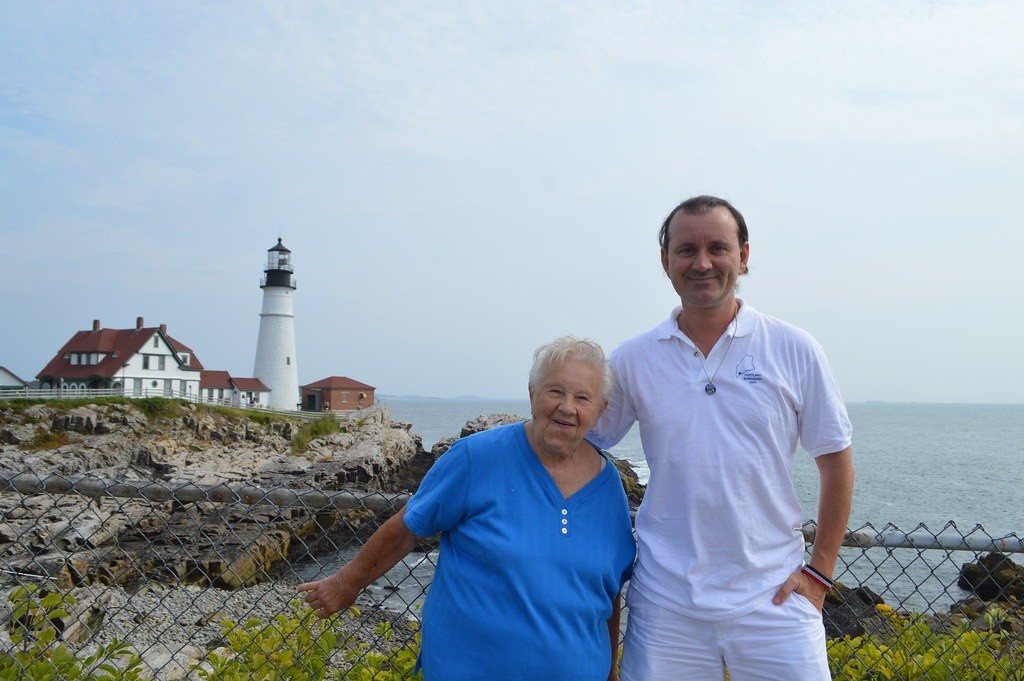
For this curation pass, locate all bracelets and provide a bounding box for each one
[801,563,834,591]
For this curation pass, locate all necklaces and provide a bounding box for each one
[684,306,738,394]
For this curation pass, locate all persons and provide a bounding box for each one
[584,196,855,681]
[297,335,636,681]
